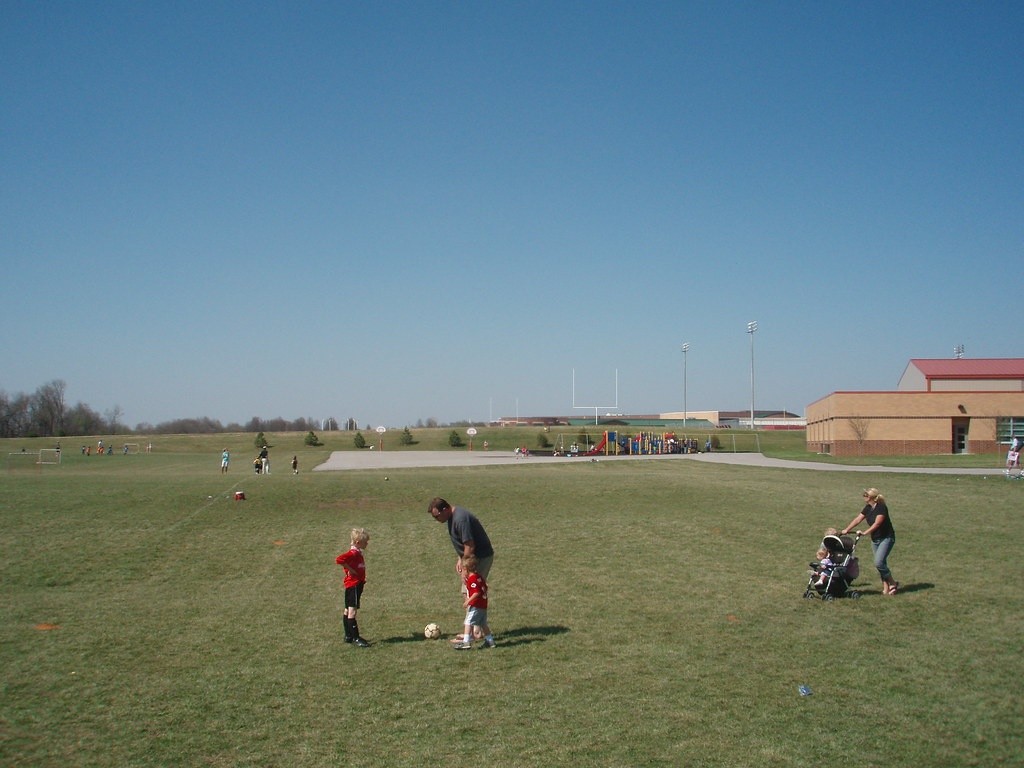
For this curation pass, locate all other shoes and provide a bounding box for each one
[1003,469,1010,475]
[814,580,823,586]
[345,636,355,642]
[353,636,369,647]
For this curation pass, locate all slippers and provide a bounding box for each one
[890,581,899,595]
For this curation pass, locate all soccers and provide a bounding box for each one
[385,477,389,481]
[425,623,441,640]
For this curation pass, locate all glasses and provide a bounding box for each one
[434,512,441,519]
[863,494,869,498]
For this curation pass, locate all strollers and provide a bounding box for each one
[797,531,861,603]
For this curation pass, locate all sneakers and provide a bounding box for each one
[478,640,496,649]
[455,642,472,649]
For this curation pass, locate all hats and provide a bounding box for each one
[263,446,267,449]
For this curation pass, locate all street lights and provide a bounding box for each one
[322,419,331,431]
[681,342,691,427]
[744,320,761,430]
[347,418,359,431]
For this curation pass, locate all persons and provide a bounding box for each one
[82,445,85,455]
[816,528,838,565]
[428,497,494,639]
[86,446,91,456]
[484,440,488,450]
[124,445,128,454]
[591,443,595,451]
[96,440,113,455]
[1003,435,1024,474]
[257,446,271,474]
[521,445,527,457]
[704,440,711,451]
[813,550,833,585]
[454,557,497,650]
[514,446,520,460]
[842,488,899,595]
[335,529,371,648]
[221,447,230,475]
[291,456,298,475]
[253,456,262,475]
